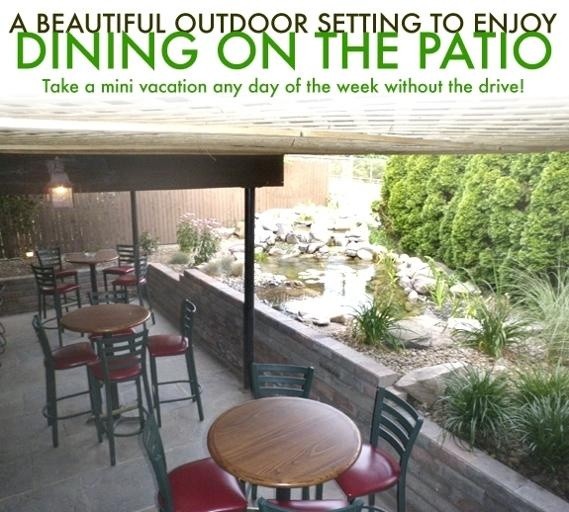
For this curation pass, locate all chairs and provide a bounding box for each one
[30,244,422,512]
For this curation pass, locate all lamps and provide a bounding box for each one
[47,155,77,210]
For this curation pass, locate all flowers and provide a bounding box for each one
[177,212,222,267]
